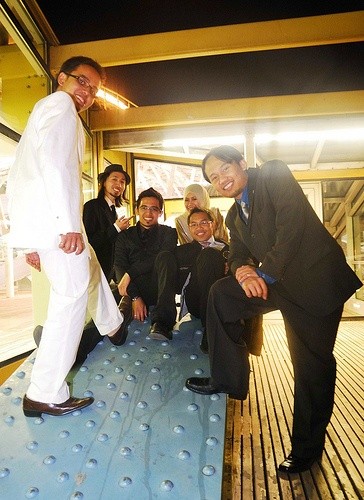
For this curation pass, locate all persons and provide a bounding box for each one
[175,184,229,244]
[33,187,178,367]
[149,207,232,354]
[185,145,363,472]
[6,56,133,418]
[82,164,134,281]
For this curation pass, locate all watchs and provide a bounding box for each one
[132,296,139,300]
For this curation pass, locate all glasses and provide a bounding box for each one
[139,206,163,213]
[188,221,213,228]
[66,73,100,99]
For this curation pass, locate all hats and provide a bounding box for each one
[96,164,130,185]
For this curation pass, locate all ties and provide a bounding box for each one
[240,201,264,266]
[201,241,212,251]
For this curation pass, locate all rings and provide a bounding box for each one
[72,245,77,248]
[246,274,250,277]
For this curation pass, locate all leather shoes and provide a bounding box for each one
[185,377,247,401]
[108,295,133,346]
[279,451,321,473]
[148,321,174,340]
[33,325,88,369]
[23,394,94,418]
